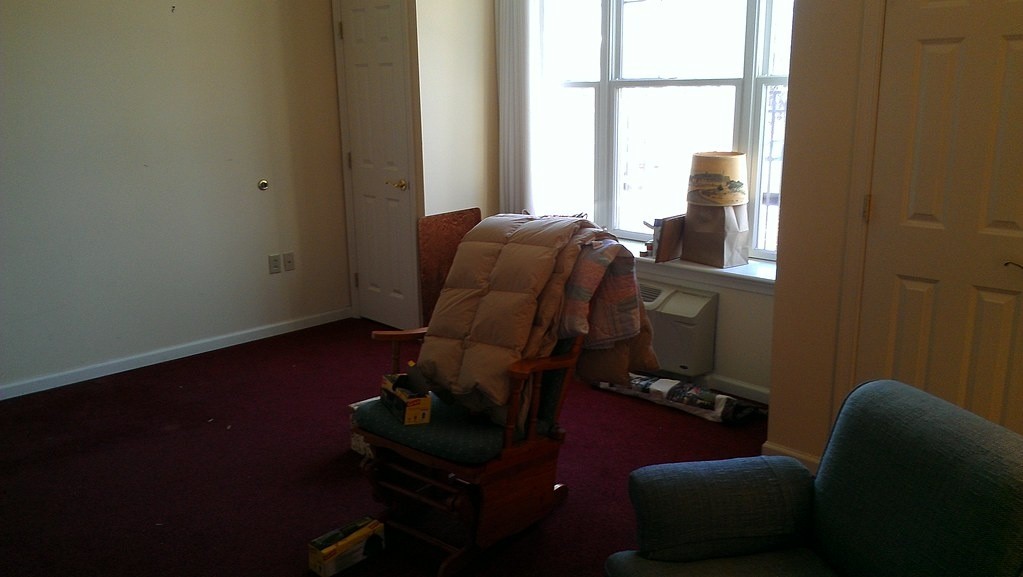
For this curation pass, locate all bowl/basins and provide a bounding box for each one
[686,151,749,207]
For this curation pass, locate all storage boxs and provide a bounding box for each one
[307,515,384,577]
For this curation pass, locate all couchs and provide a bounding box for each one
[606,381,1022,577]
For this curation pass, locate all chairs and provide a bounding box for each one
[343,292,598,557]
